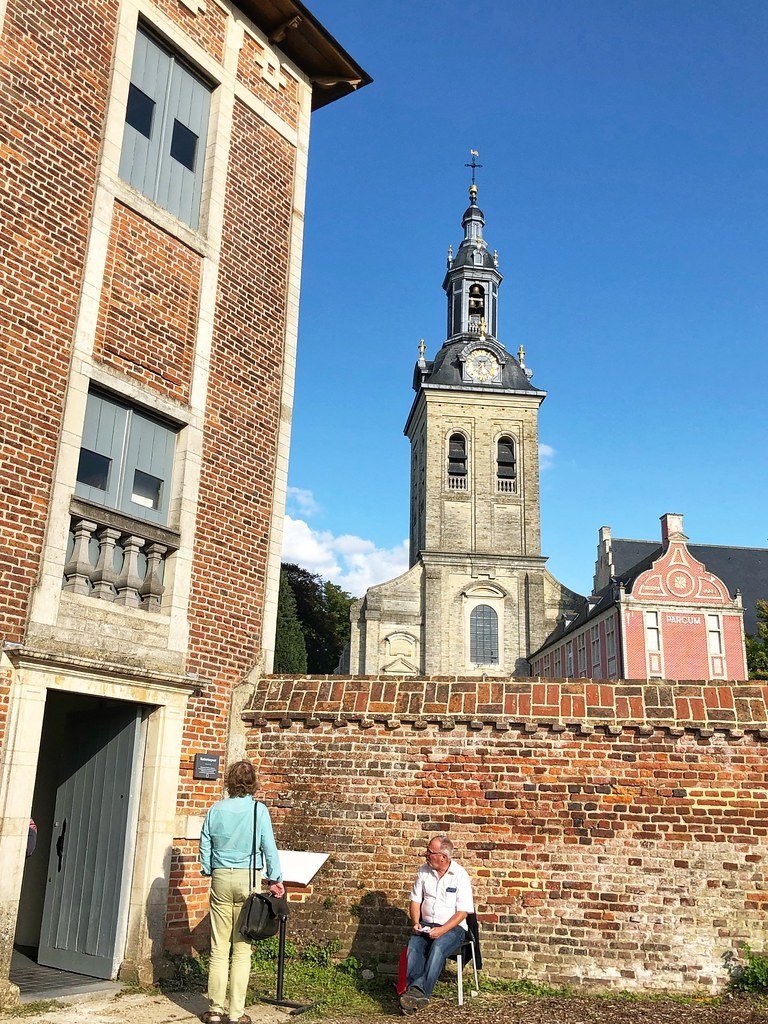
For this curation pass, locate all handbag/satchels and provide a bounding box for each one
[238,892,290,940]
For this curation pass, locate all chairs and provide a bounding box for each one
[451,907,483,1005]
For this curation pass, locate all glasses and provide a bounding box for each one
[427,850,441,855]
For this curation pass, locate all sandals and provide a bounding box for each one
[201,1011,222,1024]
[226,1013,252,1024]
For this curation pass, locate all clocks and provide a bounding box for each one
[462,350,503,386]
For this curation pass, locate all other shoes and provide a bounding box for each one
[399,988,429,1018]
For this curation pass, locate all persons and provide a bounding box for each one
[197,760,286,1023]
[398,835,474,1017]
